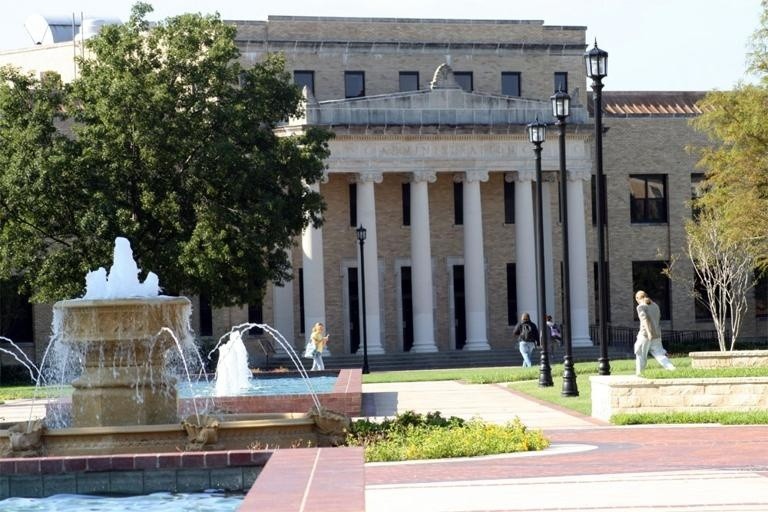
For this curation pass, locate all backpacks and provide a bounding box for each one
[519,321,533,341]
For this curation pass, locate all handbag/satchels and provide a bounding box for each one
[550,325,562,340]
[304,343,318,359]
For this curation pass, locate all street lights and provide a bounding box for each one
[525,117,555,387]
[583,37,611,375]
[550,82,579,396]
[355,223,370,374]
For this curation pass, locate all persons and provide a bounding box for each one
[310,322,328,370]
[633,289,677,376]
[513,313,540,367]
[546,315,557,363]
